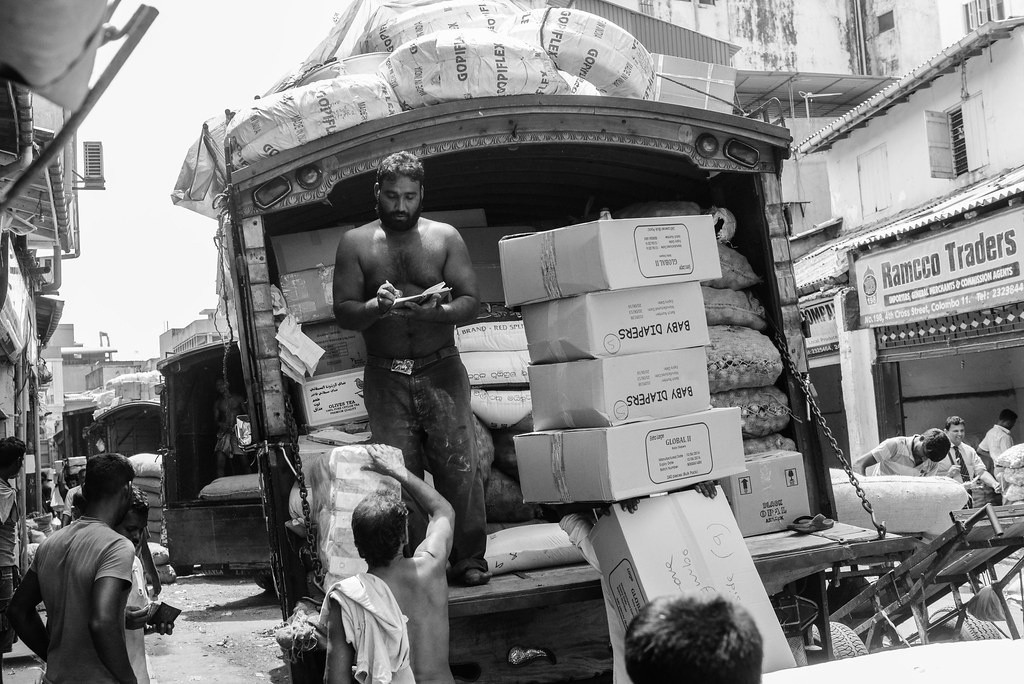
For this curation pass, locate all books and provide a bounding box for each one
[380,281,453,320]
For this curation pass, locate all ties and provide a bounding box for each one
[953,446,973,497]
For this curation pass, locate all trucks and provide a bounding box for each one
[52,93,932,684]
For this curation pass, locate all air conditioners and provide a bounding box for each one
[82,141,104,187]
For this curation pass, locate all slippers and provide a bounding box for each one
[786,513,834,533]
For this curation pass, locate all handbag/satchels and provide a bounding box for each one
[968,584,1006,621]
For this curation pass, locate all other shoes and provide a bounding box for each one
[458,567,490,586]
[967,577,983,588]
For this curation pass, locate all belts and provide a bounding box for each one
[364,346,459,375]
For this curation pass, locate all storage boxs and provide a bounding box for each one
[648,51,738,115]
[269,206,811,675]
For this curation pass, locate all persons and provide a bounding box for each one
[5,453,175,684]
[326,443,456,684]
[212,373,251,478]
[936,416,1004,594]
[853,428,951,476]
[624,593,765,684]
[0,436,37,684]
[977,409,1018,506]
[333,151,493,586]
